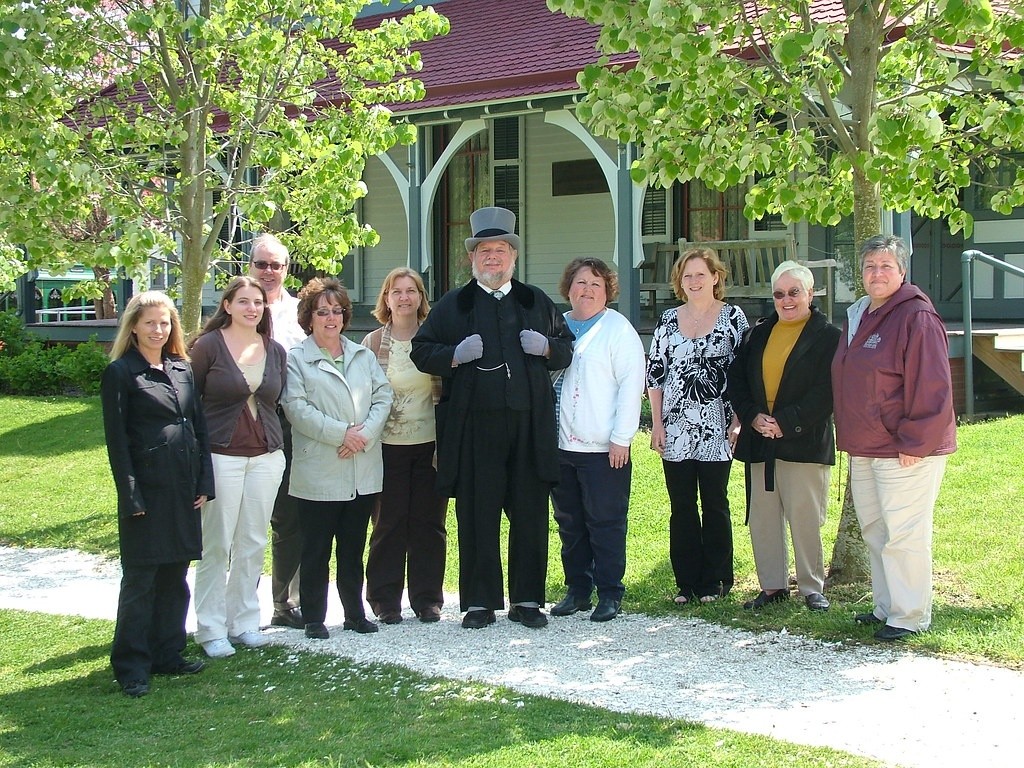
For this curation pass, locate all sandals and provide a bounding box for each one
[675,590,695,605]
[700,594,723,604]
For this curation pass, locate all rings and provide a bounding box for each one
[619,457,624,460]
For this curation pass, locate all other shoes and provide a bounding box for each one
[378,610,403,623]
[419,606,440,621]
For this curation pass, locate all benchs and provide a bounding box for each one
[678,235,837,324]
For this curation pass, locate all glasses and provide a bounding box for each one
[252,260,287,270]
[312,308,346,316]
[772,288,808,299]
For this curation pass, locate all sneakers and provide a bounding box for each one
[200,637,236,658]
[228,631,270,647]
[114,673,147,695]
[152,662,204,674]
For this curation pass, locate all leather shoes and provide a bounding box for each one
[271,607,303,628]
[550,585,590,614]
[304,622,329,638]
[873,626,917,640]
[744,588,790,609]
[805,592,830,612]
[855,612,884,624]
[343,618,379,633]
[590,598,621,621]
[462,609,495,628]
[508,604,547,626]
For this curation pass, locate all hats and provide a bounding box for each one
[464,207,519,254]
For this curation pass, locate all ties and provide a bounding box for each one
[493,291,503,300]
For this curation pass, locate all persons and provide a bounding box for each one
[360,267,450,625]
[723,259,842,610]
[543,256,646,622]
[247,235,309,629]
[99,291,216,698]
[831,234,957,642]
[411,208,575,629]
[646,248,752,606]
[279,276,393,638]
[189,276,288,658]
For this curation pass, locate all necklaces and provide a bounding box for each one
[571,306,605,334]
[685,299,714,325]
[393,325,417,353]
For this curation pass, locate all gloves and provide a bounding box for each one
[520,329,548,357]
[453,335,482,364]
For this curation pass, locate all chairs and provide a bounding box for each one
[640,242,678,319]
